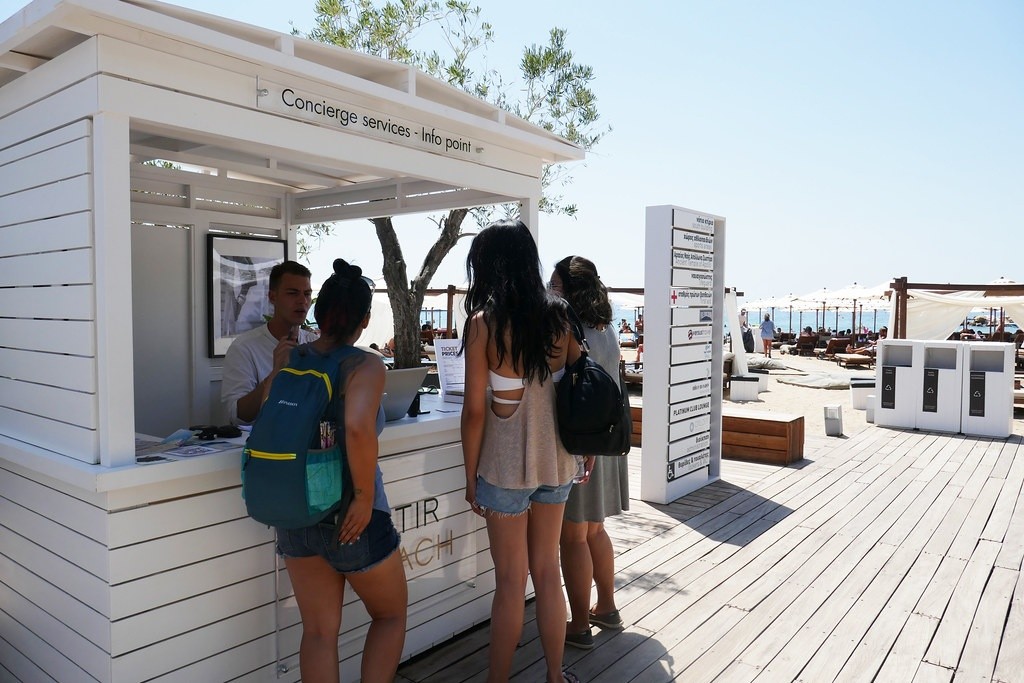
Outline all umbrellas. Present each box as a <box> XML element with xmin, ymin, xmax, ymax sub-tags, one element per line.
<box><xmin>743</xmin><ymin>278</ymin><xmax>919</xmax><ymax>350</ymax></box>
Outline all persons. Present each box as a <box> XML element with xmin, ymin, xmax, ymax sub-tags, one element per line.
<box><xmin>845</xmin><ymin>326</ymin><xmax>888</xmax><ymax>353</ymax></box>
<box><xmin>369</xmin><ymin>343</ymin><xmax>394</xmax><ymax>357</ymax></box>
<box><xmin>960</xmin><ymin>324</ymin><xmax>1001</xmax><ymax>340</ymax></box>
<box><xmin>739</xmin><ymin>308</ymin><xmax>748</xmax><ymax>333</ymax></box>
<box><xmin>459</xmin><ymin>217</ymin><xmax>594</xmax><ymax>683</ymax></box>
<box><xmin>273</xmin><ymin>258</ymin><xmax>409</xmax><ymax>683</ymax></box>
<box><xmin>421</xmin><ymin>325</ymin><xmax>436</xmax><ymax>346</ymax></box>
<box><xmin>777</xmin><ymin>328</ymin><xmax>784</xmax><ymax>337</ymax></box>
<box><xmin>619</xmin><ymin>315</ymin><xmax>642</xmax><ymax>333</ymax></box>
<box><xmin>760</xmin><ymin>313</ymin><xmax>775</xmax><ymax>358</ymax></box>
<box><xmin>219</xmin><ymin>261</ymin><xmax>318</xmax><ymax>428</ymax></box>
<box><xmin>545</xmin><ymin>255</ymin><xmax>630</xmax><ymax>650</ymax></box>
<box><xmin>801</xmin><ymin>326</ymin><xmax>815</xmax><ymax>337</ymax></box>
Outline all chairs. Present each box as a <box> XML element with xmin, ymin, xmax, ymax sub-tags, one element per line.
<box><xmin>772</xmin><ymin>332</ymin><xmax>877</xmax><ymax>368</ymax></box>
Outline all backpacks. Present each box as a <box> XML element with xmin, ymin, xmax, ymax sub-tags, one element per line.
<box><xmin>240</xmin><ymin>345</ymin><xmax>362</xmax><ymax>529</ymax></box>
<box><xmin>555</xmin><ymin>296</ymin><xmax>631</xmax><ymax>456</ymax></box>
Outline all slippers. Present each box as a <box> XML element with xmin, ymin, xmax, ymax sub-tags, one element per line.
<box><xmin>561</xmin><ymin>670</ymin><xmax>580</xmax><ymax>683</ymax></box>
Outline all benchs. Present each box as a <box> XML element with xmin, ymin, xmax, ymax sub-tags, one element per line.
<box><xmin>630</xmin><ymin>401</ymin><xmax>804</xmax><ymax>466</ymax></box>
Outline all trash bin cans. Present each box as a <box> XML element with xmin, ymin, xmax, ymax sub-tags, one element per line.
<box><xmin>874</xmin><ymin>338</ymin><xmax>1016</xmax><ymax>439</ymax></box>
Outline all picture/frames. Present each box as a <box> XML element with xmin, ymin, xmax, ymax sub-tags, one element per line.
<box><xmin>207</xmin><ymin>233</ymin><xmax>288</xmax><ymax>358</ymax></box>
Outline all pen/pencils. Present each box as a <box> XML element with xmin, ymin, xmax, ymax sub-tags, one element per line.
<box><xmin>287</xmin><ymin>325</ymin><xmax>294</xmax><ymax>340</ymax></box>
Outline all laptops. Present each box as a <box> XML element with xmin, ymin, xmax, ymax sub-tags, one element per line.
<box><xmin>381</xmin><ymin>367</ymin><xmax>430</xmax><ymax>422</ymax></box>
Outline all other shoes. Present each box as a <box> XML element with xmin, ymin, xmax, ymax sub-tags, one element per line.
<box><xmin>564</xmin><ymin>619</ymin><xmax>595</xmax><ymax>648</ymax></box>
<box><xmin>586</xmin><ymin>604</ymin><xmax>624</xmax><ymax>628</ymax></box>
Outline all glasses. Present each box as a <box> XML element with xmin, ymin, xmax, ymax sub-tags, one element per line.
<box><xmin>548</xmin><ymin>282</ymin><xmax>563</xmax><ymax>291</ymax></box>
<box><xmin>360</xmin><ymin>276</ymin><xmax>375</xmax><ymax>320</ymax></box>
